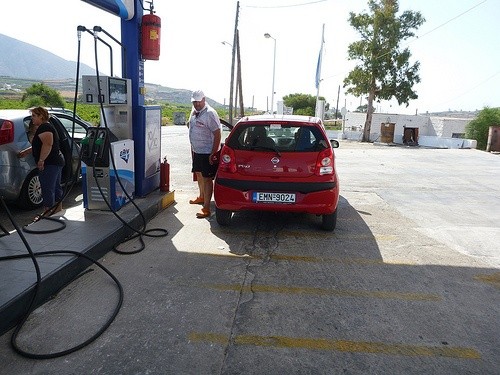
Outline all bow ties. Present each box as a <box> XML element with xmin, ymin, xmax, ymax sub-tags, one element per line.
<box><xmin>192</xmin><ymin>110</ymin><xmax>200</xmax><ymax>117</ymax></box>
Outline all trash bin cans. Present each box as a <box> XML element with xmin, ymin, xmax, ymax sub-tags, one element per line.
<box><xmin>381</xmin><ymin>123</ymin><xmax>396</xmax><ymax>143</ymax></box>
<box><xmin>403</xmin><ymin>124</ymin><xmax>419</xmax><ymax>147</ymax></box>
<box><xmin>174</xmin><ymin>112</ymin><xmax>186</xmax><ymax>125</ymax></box>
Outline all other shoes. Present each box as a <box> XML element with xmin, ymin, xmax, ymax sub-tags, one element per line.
<box><xmin>35</xmin><ymin>207</ymin><xmax>51</xmax><ymax>220</ymax></box>
<box><xmin>54</xmin><ymin>202</ymin><xmax>62</xmax><ymax>212</ymax></box>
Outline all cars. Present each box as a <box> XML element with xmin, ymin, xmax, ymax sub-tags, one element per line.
<box><xmin>192</xmin><ymin>114</ymin><xmax>341</xmax><ymax>231</ymax></box>
<box><xmin>0</xmin><ymin>106</ymin><xmax>93</xmax><ymax>209</ymax></box>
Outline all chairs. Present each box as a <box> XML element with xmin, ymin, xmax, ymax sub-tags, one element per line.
<box><xmin>245</xmin><ymin>128</ymin><xmax>275</xmax><ymax>148</ymax></box>
<box><xmin>289</xmin><ymin>127</ymin><xmax>316</xmax><ymax>148</ymax></box>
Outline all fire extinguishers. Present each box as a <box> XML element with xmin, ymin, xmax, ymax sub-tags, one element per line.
<box><xmin>160</xmin><ymin>155</ymin><xmax>169</xmax><ymax>192</ymax></box>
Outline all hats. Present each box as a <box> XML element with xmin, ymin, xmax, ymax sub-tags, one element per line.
<box><xmin>190</xmin><ymin>90</ymin><xmax>205</xmax><ymax>102</ymax></box>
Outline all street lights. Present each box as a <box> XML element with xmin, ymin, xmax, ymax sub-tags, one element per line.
<box><xmin>222</xmin><ymin>40</ymin><xmax>238</xmax><ymax>117</ymax></box>
<box><xmin>264</xmin><ymin>33</ymin><xmax>277</xmax><ymax>114</ymax></box>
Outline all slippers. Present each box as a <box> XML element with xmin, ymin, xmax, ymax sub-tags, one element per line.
<box><xmin>189</xmin><ymin>197</ymin><xmax>204</xmax><ymax>204</ymax></box>
<box><xmin>196</xmin><ymin>208</ymin><xmax>211</xmax><ymax>217</ymax></box>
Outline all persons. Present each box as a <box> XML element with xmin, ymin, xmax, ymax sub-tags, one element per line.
<box><xmin>26</xmin><ymin>120</ymin><xmax>38</xmax><ymax>143</ymax></box>
<box><xmin>30</xmin><ymin>106</ymin><xmax>65</xmax><ymax>221</ymax></box>
<box><xmin>188</xmin><ymin>89</ymin><xmax>222</xmax><ymax>217</ymax></box>
